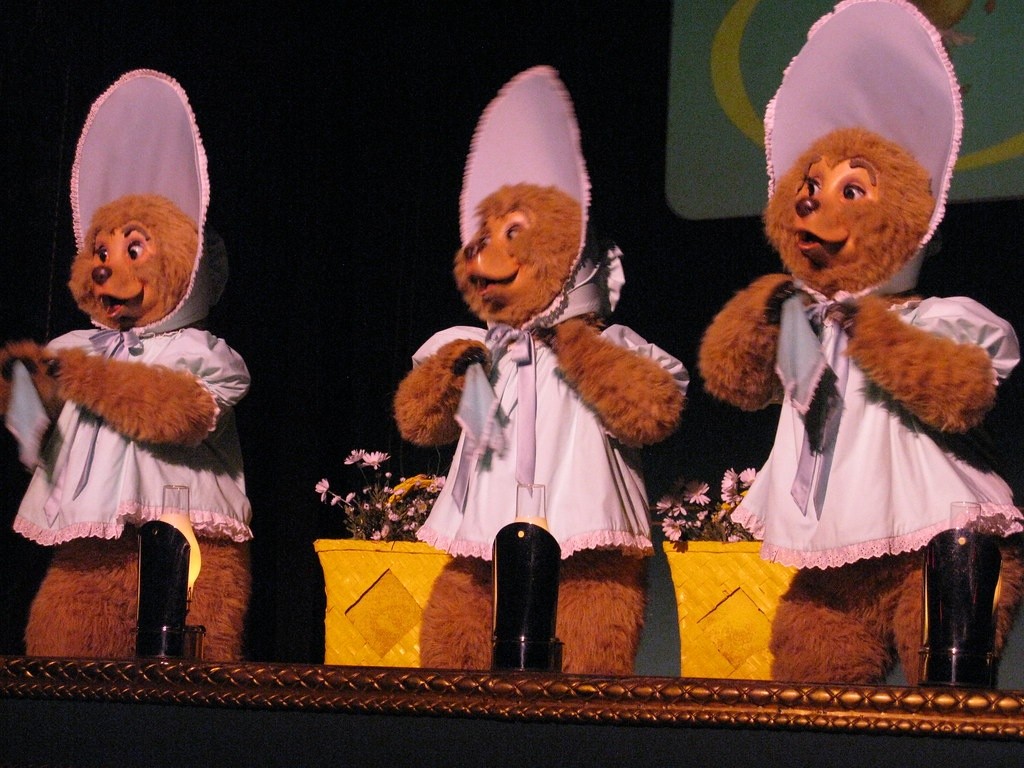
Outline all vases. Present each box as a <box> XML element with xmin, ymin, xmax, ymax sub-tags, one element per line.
<box><xmin>313</xmin><ymin>539</ymin><xmax>491</xmax><ymax>670</ymax></box>
<box><xmin>663</xmin><ymin>540</ymin><xmax>799</xmax><ymax>682</ymax></box>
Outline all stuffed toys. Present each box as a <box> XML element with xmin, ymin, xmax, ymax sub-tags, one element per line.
<box><xmin>0</xmin><ymin>70</ymin><xmax>254</xmax><ymax>671</ymax></box>
<box><xmin>395</xmin><ymin>63</ymin><xmax>690</xmax><ymax>674</ymax></box>
<box><xmin>699</xmin><ymin>1</ymin><xmax>1022</xmax><ymax>688</ymax></box>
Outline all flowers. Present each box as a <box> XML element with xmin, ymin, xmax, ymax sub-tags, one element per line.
<box><xmin>315</xmin><ymin>448</ymin><xmax>446</xmax><ymax>541</ymax></box>
<box><xmin>655</xmin><ymin>461</ymin><xmax>756</xmax><ymax>544</ymax></box>
